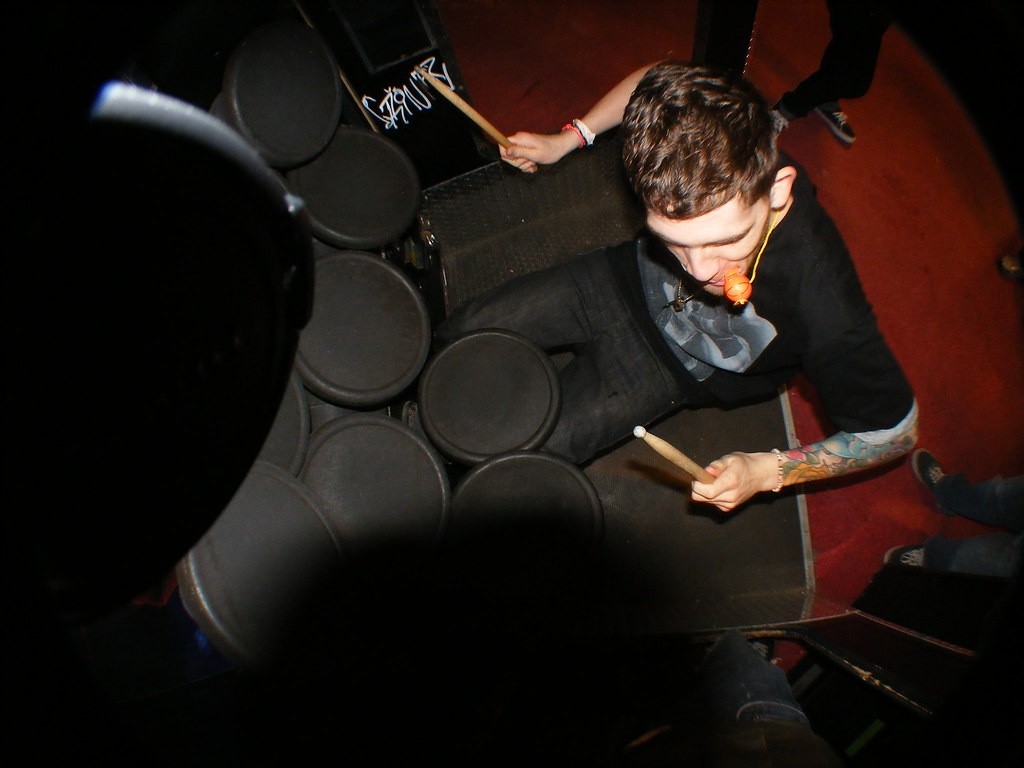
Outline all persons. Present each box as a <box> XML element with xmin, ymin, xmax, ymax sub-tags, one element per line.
<box><xmin>767</xmin><ymin>0</ymin><xmax>889</xmax><ymax>143</ymax></box>
<box><xmin>438</xmin><ymin>60</ymin><xmax>919</xmax><ymax>511</ymax></box>
<box><xmin>884</xmin><ymin>449</ymin><xmax>1024</xmax><ymax>578</ymax></box>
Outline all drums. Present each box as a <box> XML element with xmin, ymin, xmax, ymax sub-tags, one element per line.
<box><xmin>171</xmin><ymin>21</ymin><xmax>608</xmax><ymax>678</ymax></box>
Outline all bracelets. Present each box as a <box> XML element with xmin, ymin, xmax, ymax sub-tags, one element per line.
<box><xmin>563</xmin><ymin>119</ymin><xmax>596</xmax><ymax>148</ymax></box>
<box><xmin>771</xmin><ymin>449</ymin><xmax>783</xmax><ymax>492</ymax></box>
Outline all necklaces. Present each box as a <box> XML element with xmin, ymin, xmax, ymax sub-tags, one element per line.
<box><xmin>675</xmin><ymin>270</ymin><xmax>700</xmax><ymax>308</ymax></box>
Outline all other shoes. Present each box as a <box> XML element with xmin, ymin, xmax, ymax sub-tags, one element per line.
<box><xmin>997</xmin><ymin>252</ymin><xmax>1023</xmax><ymax>278</ymax></box>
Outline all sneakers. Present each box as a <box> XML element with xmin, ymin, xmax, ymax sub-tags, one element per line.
<box><xmin>819</xmin><ymin>103</ymin><xmax>855</xmax><ymax>144</ymax></box>
<box><xmin>770</xmin><ymin>98</ymin><xmax>789</xmax><ymax>140</ymax></box>
<box><xmin>883</xmin><ymin>544</ymin><xmax>923</xmax><ymax>568</ymax></box>
<box><xmin>912</xmin><ymin>448</ymin><xmax>956</xmax><ymax>515</ymax></box>
